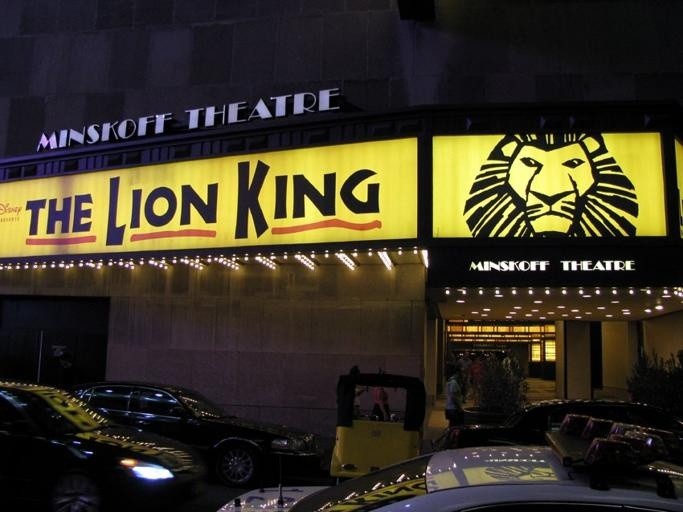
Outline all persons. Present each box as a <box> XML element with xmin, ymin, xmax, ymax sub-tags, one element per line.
<box><xmin>348</xmin><ymin>364</ymin><xmax>367</xmax><ymax>420</ymax></box>
<box><xmin>454</xmin><ymin>351</ymin><xmax>520</xmax><ymax>406</ymax></box>
<box><xmin>443</xmin><ymin>363</ymin><xmax>466</xmax><ymax>430</ymax></box>
<box><xmin>370</xmin><ymin>369</ymin><xmax>393</xmax><ymax>421</ymax></box>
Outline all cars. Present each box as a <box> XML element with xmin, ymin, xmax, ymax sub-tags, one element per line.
<box><xmin>66</xmin><ymin>381</ymin><xmax>325</xmax><ymax>489</ymax></box>
<box><xmin>214</xmin><ymin>412</ymin><xmax>683</xmax><ymax>512</ymax></box>
<box><xmin>429</xmin><ymin>398</ymin><xmax>683</xmax><ymax>468</ymax></box>
<box><xmin>0</xmin><ymin>380</ymin><xmax>208</xmax><ymax>512</ymax></box>
